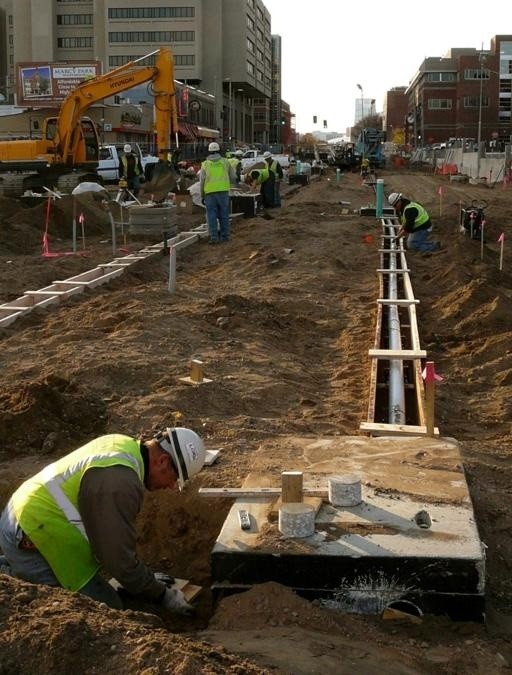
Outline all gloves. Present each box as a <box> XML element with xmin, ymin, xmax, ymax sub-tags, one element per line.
<box><xmin>153</xmin><ymin>572</ymin><xmax>197</xmax><ymax>616</ymax></box>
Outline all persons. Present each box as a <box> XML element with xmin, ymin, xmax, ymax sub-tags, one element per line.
<box><xmin>0</xmin><ymin>425</ymin><xmax>207</xmax><ymax>624</ymax></box>
<box><xmin>118</xmin><ymin>144</ymin><xmax>145</xmax><ymax>201</ymax></box>
<box><xmin>199</xmin><ymin>142</ymin><xmax>238</xmax><ymax>245</ymax></box>
<box><xmin>387</xmin><ymin>192</ymin><xmax>441</xmax><ymax>253</ymax></box>
<box><xmin>219</xmin><ymin>139</ymin><xmax>285</xmax><ymax>210</ymax></box>
<box><xmin>142</xmin><ymin>150</ymin><xmax>203</xmax><ymax>180</ymax></box>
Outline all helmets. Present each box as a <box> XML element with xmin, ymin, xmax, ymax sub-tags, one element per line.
<box><xmin>123</xmin><ymin>144</ymin><xmax>132</xmax><ymax>153</ymax></box>
<box><xmin>235</xmin><ymin>150</ymin><xmax>244</xmax><ymax>157</ymax></box>
<box><xmin>208</xmin><ymin>142</ymin><xmax>220</xmax><ymax>152</ymax></box>
<box><xmin>263</xmin><ymin>151</ymin><xmax>273</xmax><ymax>159</ymax></box>
<box><xmin>164</xmin><ymin>426</ymin><xmax>206</xmax><ymax>494</ymax></box>
<box><xmin>388</xmin><ymin>192</ymin><xmax>403</xmax><ymax>206</ymax></box>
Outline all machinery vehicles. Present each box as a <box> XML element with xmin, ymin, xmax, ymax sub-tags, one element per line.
<box><xmin>0</xmin><ymin>47</ymin><xmax>181</xmax><ymax>195</ymax></box>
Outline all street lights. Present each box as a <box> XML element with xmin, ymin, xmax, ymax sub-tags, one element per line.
<box><xmin>476</xmin><ymin>51</ymin><xmax>489</xmax><ymax>180</ymax></box>
<box><xmin>357</xmin><ymin>85</ymin><xmax>365</xmax><ymax>161</ymax></box>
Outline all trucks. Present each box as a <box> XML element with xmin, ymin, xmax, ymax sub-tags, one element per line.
<box><xmin>96</xmin><ymin>144</ymin><xmax>159</xmax><ymax>183</ymax></box>
<box><xmin>240</xmin><ymin>150</ymin><xmax>290</xmax><ymax>172</ymax></box>
